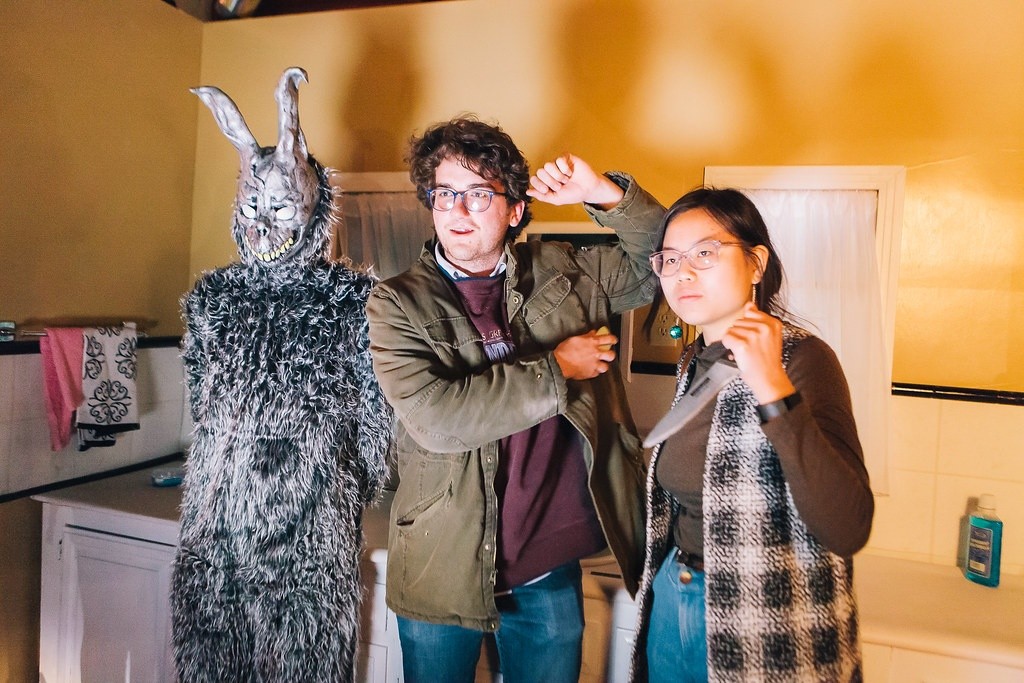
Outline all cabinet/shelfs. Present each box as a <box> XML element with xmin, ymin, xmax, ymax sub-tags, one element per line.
<box><xmin>35</xmin><ymin>503</ymin><xmax>1024</xmax><ymax>683</ymax></box>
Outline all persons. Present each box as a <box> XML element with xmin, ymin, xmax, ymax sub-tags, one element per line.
<box><xmin>366</xmin><ymin>114</ymin><xmax>669</xmax><ymax>683</ymax></box>
<box><xmin>626</xmin><ymin>186</ymin><xmax>875</xmax><ymax>682</ymax></box>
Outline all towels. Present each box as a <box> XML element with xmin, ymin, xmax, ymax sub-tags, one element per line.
<box><xmin>39</xmin><ymin>325</ymin><xmax>142</xmax><ymax>453</ymax></box>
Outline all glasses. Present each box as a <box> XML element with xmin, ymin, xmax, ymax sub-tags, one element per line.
<box><xmin>647</xmin><ymin>240</ymin><xmax>746</xmax><ymax>279</ymax></box>
<box><xmin>426</xmin><ymin>188</ymin><xmax>517</xmax><ymax>213</ymax></box>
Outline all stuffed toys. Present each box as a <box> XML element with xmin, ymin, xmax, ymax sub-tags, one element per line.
<box><xmin>170</xmin><ymin>65</ymin><xmax>398</xmax><ymax>683</ymax></box>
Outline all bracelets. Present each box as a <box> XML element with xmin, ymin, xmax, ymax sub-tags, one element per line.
<box><xmin>752</xmin><ymin>391</ymin><xmax>804</xmax><ymax>425</ymax></box>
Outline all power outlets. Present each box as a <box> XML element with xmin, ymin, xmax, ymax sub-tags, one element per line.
<box><xmin>649</xmin><ymin>303</ymin><xmax>679</xmax><ymax>346</ymax></box>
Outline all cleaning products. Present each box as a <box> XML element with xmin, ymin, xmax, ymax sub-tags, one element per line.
<box><xmin>962</xmin><ymin>491</ymin><xmax>1004</xmax><ymax>588</ymax></box>
